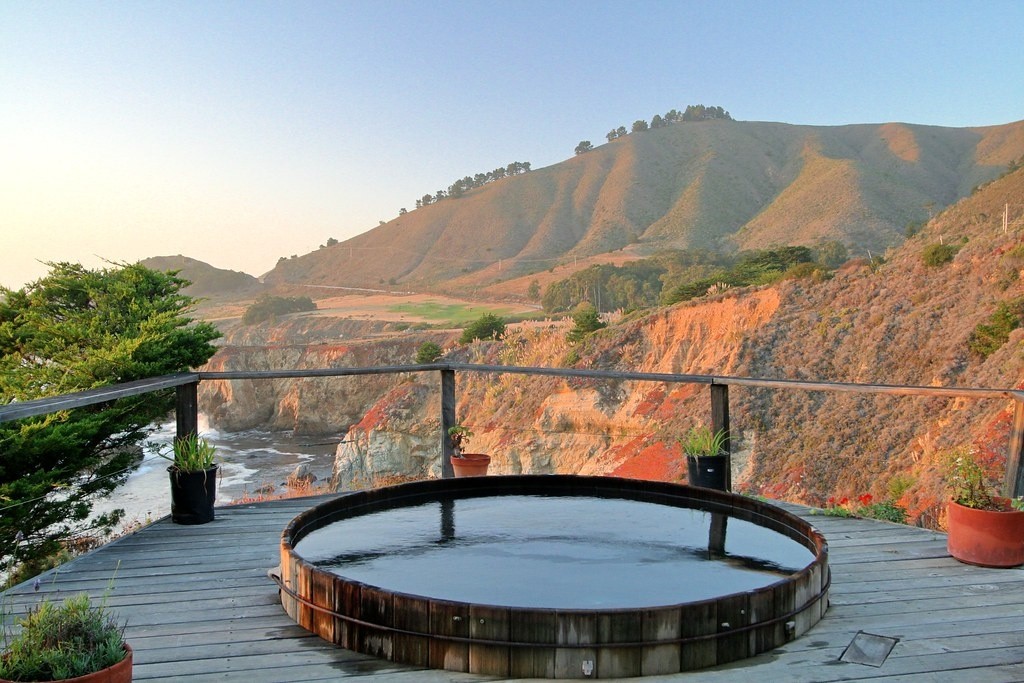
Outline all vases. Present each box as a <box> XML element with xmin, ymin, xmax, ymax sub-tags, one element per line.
<box><xmin>948</xmin><ymin>445</ymin><xmax>1024</xmax><ymax>569</ymax></box>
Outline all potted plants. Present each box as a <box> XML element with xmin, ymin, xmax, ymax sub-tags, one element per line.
<box><xmin>675</xmin><ymin>424</ymin><xmax>743</xmax><ymax>492</ymax></box>
<box><xmin>145</xmin><ymin>428</ymin><xmax>221</xmax><ymax>525</ymax></box>
<box><xmin>0</xmin><ymin>557</ymin><xmax>134</xmax><ymax>683</ymax></box>
<box><xmin>448</xmin><ymin>425</ymin><xmax>491</xmax><ymax>479</ymax></box>
